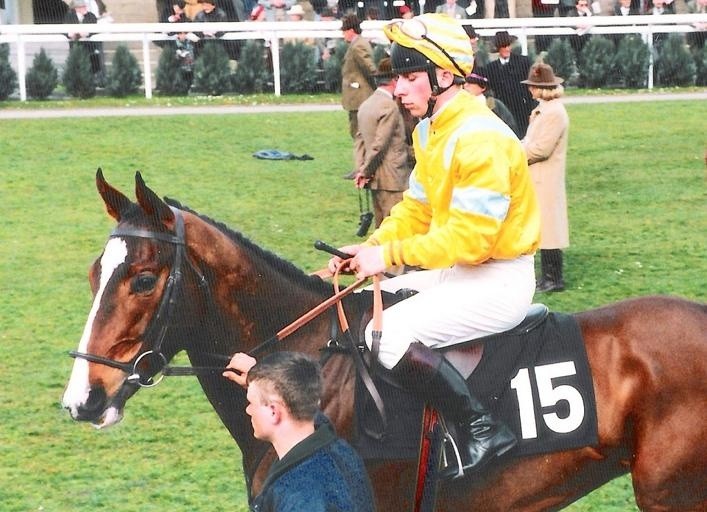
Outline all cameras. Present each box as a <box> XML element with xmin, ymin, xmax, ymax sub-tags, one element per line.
<box><xmin>356</xmin><ymin>213</ymin><xmax>374</xmax><ymax>237</ymax></box>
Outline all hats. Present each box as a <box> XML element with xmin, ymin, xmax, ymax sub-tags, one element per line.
<box><xmin>399</xmin><ymin>5</ymin><xmax>411</xmax><ymax>15</ymax></box>
<box><xmin>489</xmin><ymin>31</ymin><xmax>518</xmax><ymax>53</ymax></box>
<box><xmin>465</xmin><ymin>66</ymin><xmax>492</xmax><ymax>86</ymax></box>
<box><xmin>321</xmin><ymin>5</ymin><xmax>335</xmax><ymax>17</ymax></box>
<box><xmin>460</xmin><ymin>24</ymin><xmax>480</xmax><ymax>40</ymax></box>
<box><xmin>286</xmin><ymin>4</ymin><xmax>306</xmax><ymax>16</ymax></box>
<box><xmin>68</xmin><ymin>0</ymin><xmax>87</xmax><ymax>10</ymax></box>
<box><xmin>340</xmin><ymin>13</ymin><xmax>363</xmax><ymax>30</ymax></box>
<box><xmin>518</xmin><ymin>63</ymin><xmax>565</xmax><ymax>86</ymax></box>
<box><xmin>371</xmin><ymin>57</ymin><xmax>391</xmax><ymax>77</ymax></box>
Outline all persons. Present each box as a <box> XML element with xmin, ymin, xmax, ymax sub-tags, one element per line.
<box><xmin>223</xmin><ymin>353</ymin><xmax>380</xmax><ymax>508</ymax></box>
<box><xmin>155</xmin><ymin>0</ymin><xmax>227</xmax><ymax>71</ymax></box>
<box><xmin>645</xmin><ymin>1</ymin><xmax>707</xmax><ymax>52</ymax></box>
<box><xmin>560</xmin><ymin>0</ymin><xmax>597</xmax><ymax>54</ymax></box>
<box><xmin>438</xmin><ymin>0</ymin><xmax>467</xmax><ymax>19</ymax></box>
<box><xmin>86</xmin><ymin>1</ymin><xmax>108</xmax><ymax>82</ymax></box>
<box><xmin>341</xmin><ymin>14</ymin><xmax>378</xmax><ymax>180</ymax></box>
<box><xmin>351</xmin><ymin>58</ymin><xmax>407</xmax><ymax>229</ymax></box>
<box><xmin>61</xmin><ymin>1</ymin><xmax>100</xmax><ymax>78</ymax></box>
<box><xmin>532</xmin><ymin>0</ymin><xmax>557</xmax><ymax>54</ymax></box>
<box><xmin>399</xmin><ymin>7</ymin><xmax>412</xmax><ymax>19</ymax></box>
<box><xmin>255</xmin><ymin>1</ymin><xmax>341</xmax><ymax>67</ymax></box>
<box><xmin>486</xmin><ymin>30</ymin><xmax>534</xmax><ymax>127</ymax></box>
<box><xmin>461</xmin><ymin>24</ymin><xmax>480</xmax><ymax>88</ymax></box>
<box><xmin>464</xmin><ymin>68</ymin><xmax>516</xmax><ymax>131</ymax></box>
<box><xmin>518</xmin><ymin>63</ymin><xmax>570</xmax><ymax>294</ymax></box>
<box><xmin>613</xmin><ymin>1</ymin><xmax>637</xmax><ymax>41</ymax></box>
<box><xmin>364</xmin><ymin>7</ymin><xmax>379</xmax><ymax>25</ymax></box>
<box><xmin>329</xmin><ymin>11</ymin><xmax>540</xmax><ymax>483</ymax></box>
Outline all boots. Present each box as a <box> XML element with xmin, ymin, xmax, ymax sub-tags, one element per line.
<box><xmin>535</xmin><ymin>249</ymin><xmax>564</xmax><ymax>292</ymax></box>
<box><xmin>391</xmin><ymin>340</ymin><xmax>519</xmax><ymax>482</ymax></box>
<box><xmin>537</xmin><ymin>248</ymin><xmax>546</xmax><ymax>288</ymax></box>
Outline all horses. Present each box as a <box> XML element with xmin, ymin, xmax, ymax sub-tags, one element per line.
<box><xmin>61</xmin><ymin>165</ymin><xmax>706</xmax><ymax>512</ymax></box>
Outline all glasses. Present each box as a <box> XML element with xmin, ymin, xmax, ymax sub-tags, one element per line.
<box><xmin>577</xmin><ymin>3</ymin><xmax>588</xmax><ymax>6</ymax></box>
